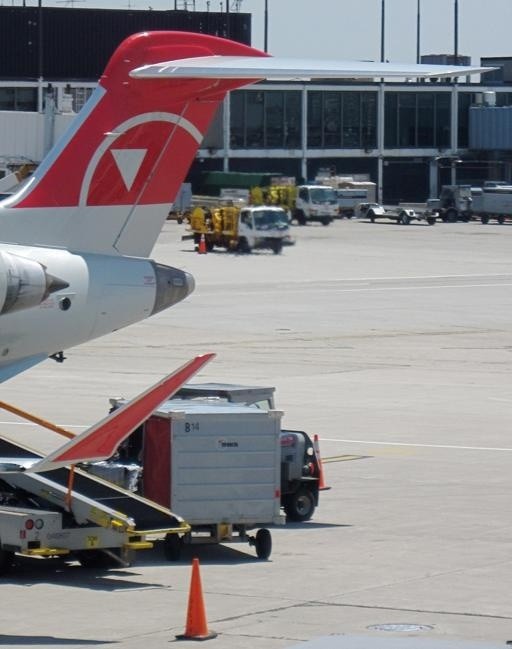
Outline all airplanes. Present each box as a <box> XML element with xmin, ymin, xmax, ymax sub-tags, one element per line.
<box><xmin>0</xmin><ymin>27</ymin><xmax>503</xmax><ymax>478</ymax></box>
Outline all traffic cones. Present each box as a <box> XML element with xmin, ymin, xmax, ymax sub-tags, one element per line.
<box><xmin>175</xmin><ymin>558</ymin><xmax>217</xmax><ymax>641</ymax></box>
<box><xmin>198</xmin><ymin>233</ymin><xmax>207</xmax><ymax>255</ymax></box>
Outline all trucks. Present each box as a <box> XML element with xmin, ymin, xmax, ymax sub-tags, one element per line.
<box><xmin>249</xmin><ymin>183</ymin><xmax>340</xmax><ymax>226</ymax></box>
<box><xmin>181</xmin><ymin>206</ymin><xmax>295</xmax><ymax>255</ymax></box>
